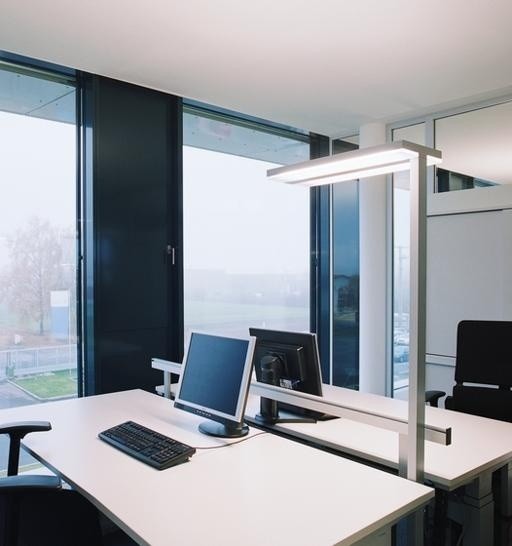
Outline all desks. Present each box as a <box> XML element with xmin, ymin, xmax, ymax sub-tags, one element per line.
<box><xmin>151</xmin><ymin>369</ymin><xmax>511</xmax><ymax>543</ymax></box>
<box><xmin>0</xmin><ymin>384</ymin><xmax>439</xmax><ymax>545</ymax></box>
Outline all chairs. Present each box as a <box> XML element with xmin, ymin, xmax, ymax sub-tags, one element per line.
<box><xmin>419</xmin><ymin>316</ymin><xmax>512</xmax><ymax>421</ymax></box>
<box><xmin>0</xmin><ymin>419</ymin><xmax>107</xmax><ymax>543</ymax></box>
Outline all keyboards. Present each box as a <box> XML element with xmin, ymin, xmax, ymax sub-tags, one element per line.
<box><xmin>98</xmin><ymin>421</ymin><xmax>196</xmax><ymax>470</ymax></box>
<box><xmin>278</xmin><ymin>402</ymin><xmax>340</xmax><ymax>421</ymax></box>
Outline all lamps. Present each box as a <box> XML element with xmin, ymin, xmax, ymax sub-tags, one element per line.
<box><xmin>261</xmin><ymin>138</ymin><xmax>442</xmax><ymax>191</ymax></box>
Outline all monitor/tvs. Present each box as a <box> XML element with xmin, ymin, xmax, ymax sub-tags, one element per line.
<box><xmin>249</xmin><ymin>328</ymin><xmax>323</xmax><ymax>423</ymax></box>
<box><xmin>174</xmin><ymin>327</ymin><xmax>257</xmax><ymax>437</ymax></box>
